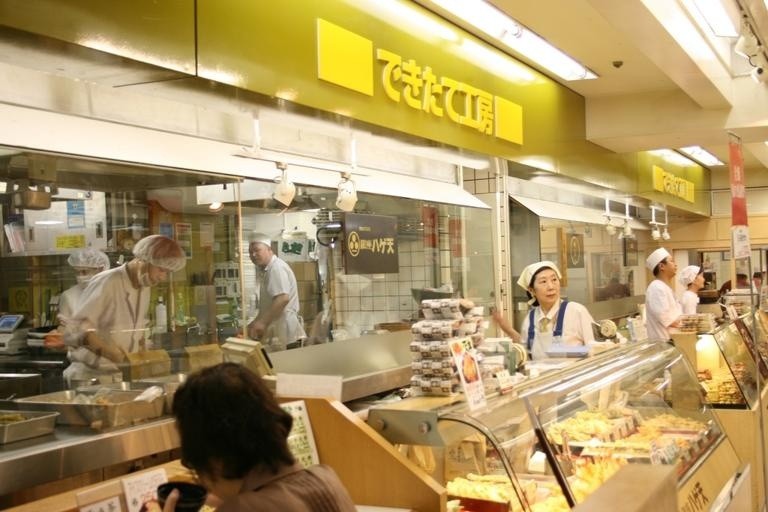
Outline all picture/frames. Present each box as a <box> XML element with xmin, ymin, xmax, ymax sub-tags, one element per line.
<box><xmin>722</xmin><ymin>251</ymin><xmax>731</xmax><ymax>261</ymax></box>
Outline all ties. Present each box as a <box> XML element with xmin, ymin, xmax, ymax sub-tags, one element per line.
<box><xmin>539</xmin><ymin>318</ymin><xmax>552</xmax><ymax>335</ymax></box>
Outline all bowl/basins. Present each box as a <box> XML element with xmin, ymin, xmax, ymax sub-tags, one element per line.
<box><xmin>155</xmin><ymin>480</ymin><xmax>204</xmax><ymax>510</ymax></box>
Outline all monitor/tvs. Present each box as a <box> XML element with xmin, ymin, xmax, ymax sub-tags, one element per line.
<box><xmin>0</xmin><ymin>314</ymin><xmax>23</xmax><ymax>331</ymax></box>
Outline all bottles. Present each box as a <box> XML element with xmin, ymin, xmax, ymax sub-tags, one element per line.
<box><xmin>155</xmin><ymin>296</ymin><xmax>169</xmax><ymax>333</ymax></box>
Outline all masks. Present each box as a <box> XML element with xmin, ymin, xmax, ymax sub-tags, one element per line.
<box><xmin>137</xmin><ymin>260</ymin><xmax>160</xmax><ymax>289</ymax></box>
<box><xmin>72</xmin><ymin>272</ymin><xmax>96</xmax><ymax>288</ymax></box>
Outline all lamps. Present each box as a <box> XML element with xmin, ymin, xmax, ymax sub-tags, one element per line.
<box><xmin>600</xmin><ymin>185</ymin><xmax>636</xmax><ymax>239</ymax></box>
<box><xmin>233</xmin><ymin>153</ymin><xmax>371</xmax><ymax>213</ymax></box>
<box><xmin>647</xmin><ymin>205</ymin><xmax>674</xmax><ymax>242</ymax></box>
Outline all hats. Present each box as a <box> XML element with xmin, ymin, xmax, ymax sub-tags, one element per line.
<box><xmin>643</xmin><ymin>244</ymin><xmax>671</xmax><ymax>274</ymax></box>
<box><xmin>677</xmin><ymin>263</ymin><xmax>703</xmax><ymax>289</ymax></box>
<box><xmin>515</xmin><ymin>259</ymin><xmax>562</xmax><ymax>291</ymax></box>
<box><xmin>247</xmin><ymin>231</ymin><xmax>273</xmax><ymax>248</ymax></box>
<box><xmin>753</xmin><ymin>264</ymin><xmax>766</xmax><ymax>273</ymax></box>
<box><xmin>734</xmin><ymin>266</ymin><xmax>748</xmax><ymax>282</ymax></box>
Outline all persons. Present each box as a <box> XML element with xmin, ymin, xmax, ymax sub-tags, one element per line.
<box><xmin>489</xmin><ymin>261</ymin><xmax>597</xmax><ymax>361</ymax></box>
<box><xmin>48</xmin><ymin>234</ymin><xmax>186</xmax><ymax>387</ymax></box>
<box><xmin>233</xmin><ymin>233</ymin><xmax>306</xmax><ymax>349</ymax></box>
<box><xmin>143</xmin><ymin>362</ymin><xmax>357</xmax><ymax>512</ymax></box>
<box><xmin>644</xmin><ymin>246</ymin><xmax>767</xmax><ymax>345</ymax></box>
